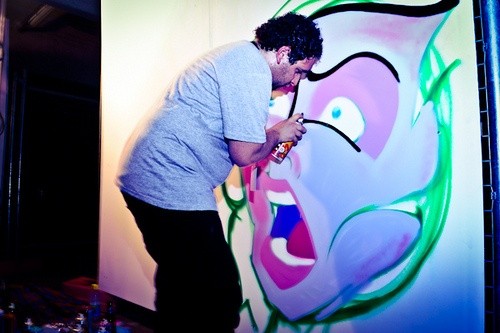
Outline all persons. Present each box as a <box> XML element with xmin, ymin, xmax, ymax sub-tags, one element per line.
<box><xmin>116</xmin><ymin>12</ymin><xmax>325</xmax><ymax>333</ymax></box>
